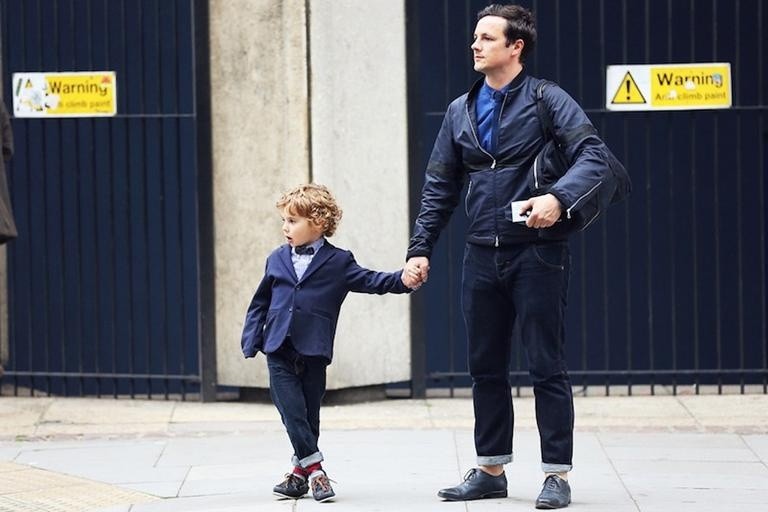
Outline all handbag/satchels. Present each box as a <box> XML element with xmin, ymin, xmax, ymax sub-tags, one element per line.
<box><xmin>528</xmin><ymin>138</ymin><xmax>632</xmax><ymax>240</ymax></box>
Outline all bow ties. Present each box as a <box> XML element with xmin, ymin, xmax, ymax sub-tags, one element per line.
<box><xmin>295</xmin><ymin>246</ymin><xmax>314</xmax><ymax>255</ymax></box>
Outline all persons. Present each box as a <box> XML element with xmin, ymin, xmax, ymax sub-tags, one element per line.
<box><xmin>403</xmin><ymin>4</ymin><xmax>610</xmax><ymax>508</ymax></box>
<box><xmin>239</xmin><ymin>181</ymin><xmax>431</xmax><ymax>503</ymax></box>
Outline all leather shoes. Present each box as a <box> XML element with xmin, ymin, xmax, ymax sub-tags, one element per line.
<box><xmin>535</xmin><ymin>474</ymin><xmax>571</xmax><ymax>509</ymax></box>
<box><xmin>312</xmin><ymin>474</ymin><xmax>336</xmax><ymax>503</ymax></box>
<box><xmin>273</xmin><ymin>472</ymin><xmax>309</xmax><ymax>498</ymax></box>
<box><xmin>438</xmin><ymin>467</ymin><xmax>508</xmax><ymax>501</ymax></box>
